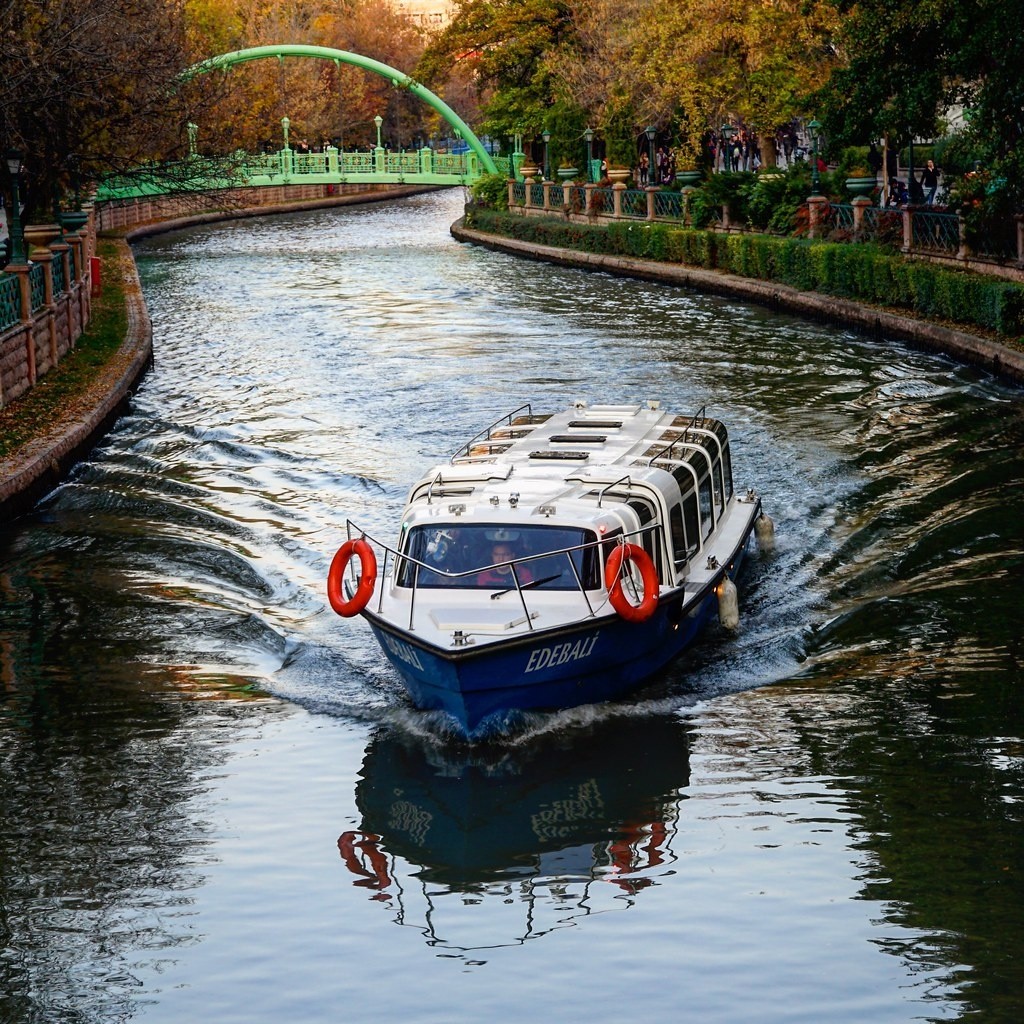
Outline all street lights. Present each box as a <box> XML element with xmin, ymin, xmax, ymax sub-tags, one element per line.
<box><xmin>280</xmin><ymin>117</ymin><xmax>291</xmax><ymax>173</ymax></box>
<box><xmin>806</xmin><ymin>121</ymin><xmax>824</xmax><ymax>197</ymax></box>
<box><xmin>187</xmin><ymin>123</ymin><xmax>198</xmax><ymax>155</ymax></box>
<box><xmin>644</xmin><ymin>126</ymin><xmax>658</xmax><ymax>186</ymax></box>
<box><xmin>541</xmin><ymin>130</ymin><xmax>551</xmax><ymax>181</ymax></box>
<box><xmin>583</xmin><ymin>129</ymin><xmax>595</xmax><ymax>184</ymax></box>
<box><xmin>374</xmin><ymin>115</ymin><xmax>383</xmax><ymax>147</ymax></box>
<box><xmin>722</xmin><ymin>124</ymin><xmax>734</xmax><ymax>173</ymax></box>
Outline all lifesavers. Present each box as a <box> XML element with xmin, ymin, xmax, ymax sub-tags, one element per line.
<box><xmin>604</xmin><ymin>542</ymin><xmax>661</xmax><ymax>624</ymax></box>
<box><xmin>326</xmin><ymin>537</ymin><xmax>378</xmax><ymax>618</ymax></box>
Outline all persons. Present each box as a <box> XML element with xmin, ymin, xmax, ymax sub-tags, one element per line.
<box><xmin>706</xmin><ymin>128</ymin><xmax>801</xmax><ymax>172</ymax></box>
<box><xmin>639</xmin><ymin>148</ymin><xmax>674</xmax><ymax>187</ymax></box>
<box><xmin>884</xmin><ymin>179</ymin><xmax>909</xmax><ymax>209</ymax></box>
<box><xmin>477</xmin><ymin>543</ymin><xmax>534</xmax><ymax>589</ymax></box>
<box><xmin>919</xmin><ymin>158</ymin><xmax>940</xmax><ymax>206</ymax></box>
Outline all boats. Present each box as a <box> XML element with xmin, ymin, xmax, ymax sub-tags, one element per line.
<box><xmin>334</xmin><ymin>716</ymin><xmax>690</xmax><ymax>962</ymax></box>
<box><xmin>328</xmin><ymin>403</ymin><xmax>775</xmax><ymax>728</ymax></box>
